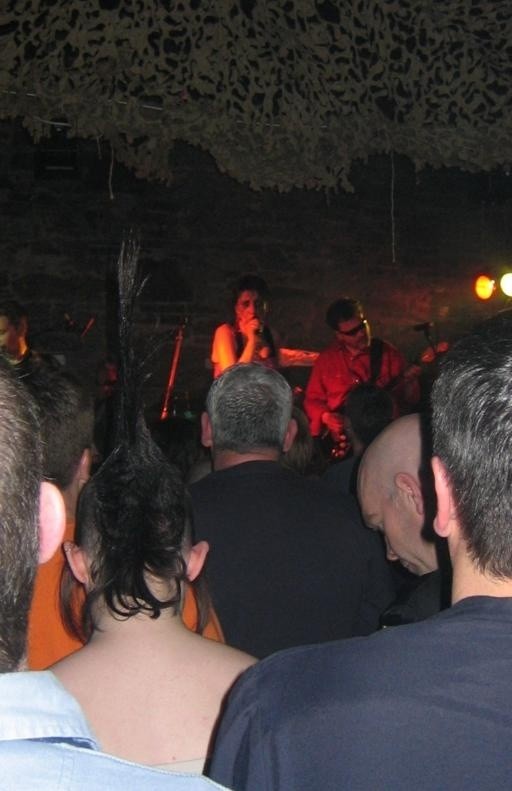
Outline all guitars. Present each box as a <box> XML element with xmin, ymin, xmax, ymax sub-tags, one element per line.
<box><xmin>318</xmin><ymin>341</ymin><xmax>448</xmax><ymax>464</ymax></box>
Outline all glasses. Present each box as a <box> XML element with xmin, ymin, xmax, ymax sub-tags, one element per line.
<box><xmin>340</xmin><ymin>318</ymin><xmax>367</xmax><ymax>336</ymax></box>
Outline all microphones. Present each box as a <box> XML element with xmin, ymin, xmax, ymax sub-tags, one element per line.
<box><xmin>412</xmin><ymin>321</ymin><xmax>435</xmax><ymax>331</ymax></box>
